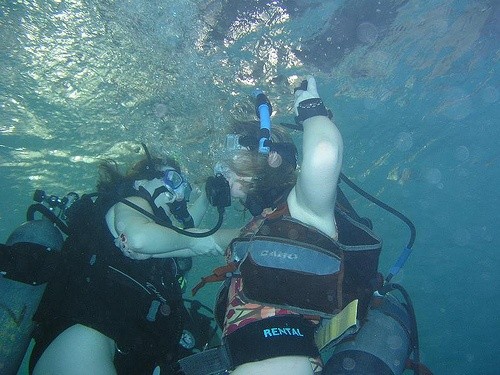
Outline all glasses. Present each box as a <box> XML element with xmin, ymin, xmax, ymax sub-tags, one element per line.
<box><xmin>157</xmin><ymin>169</ymin><xmax>193</xmax><ymax>201</ymax></box>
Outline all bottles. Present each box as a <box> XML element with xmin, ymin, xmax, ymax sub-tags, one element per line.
<box><xmin>0</xmin><ymin>191</ymin><xmax>72</xmax><ymax>375</ymax></box>
<box><xmin>333</xmin><ymin>297</ymin><xmax>414</xmax><ymax>373</ymax></box>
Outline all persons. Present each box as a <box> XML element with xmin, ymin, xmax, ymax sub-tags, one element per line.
<box><xmin>116</xmin><ymin>73</ymin><xmax>383</xmax><ymax>375</ymax></box>
<box><xmin>25</xmin><ymin>143</ymin><xmax>230</xmax><ymax>375</ymax></box>
